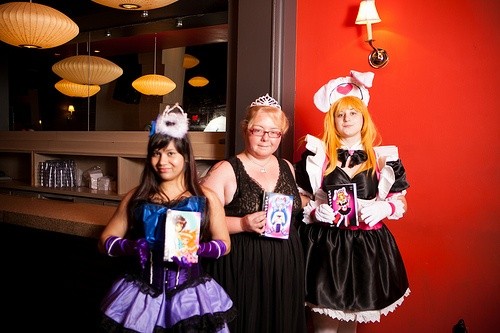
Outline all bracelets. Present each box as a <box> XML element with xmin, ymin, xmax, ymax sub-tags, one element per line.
<box><xmin>108</xmin><ymin>237</ymin><xmax>123</xmax><ymax>257</ymax></box>
<box><xmin>211</xmin><ymin>241</ymin><xmax>221</xmax><ymax>260</ymax></box>
<box><xmin>311</xmin><ymin>208</ymin><xmax>318</xmax><ymax>224</ymax></box>
<box><xmin>389</xmin><ymin>202</ymin><xmax>396</xmax><ymax>217</ymax></box>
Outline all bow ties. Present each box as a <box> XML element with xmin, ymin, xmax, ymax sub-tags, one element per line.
<box><xmin>337</xmin><ymin>149</ymin><xmax>368</xmax><ymax>168</ymax></box>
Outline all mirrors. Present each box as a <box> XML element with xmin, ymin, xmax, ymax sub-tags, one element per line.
<box><xmin>0</xmin><ymin>0</ymin><xmax>230</xmax><ymax>133</ymax></box>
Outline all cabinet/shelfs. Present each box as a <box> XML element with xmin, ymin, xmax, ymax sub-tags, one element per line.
<box><xmin>0</xmin><ymin>146</ymin><xmax>227</xmax><ymax>204</ymax></box>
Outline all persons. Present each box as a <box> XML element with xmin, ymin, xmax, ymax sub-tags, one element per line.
<box><xmin>98</xmin><ymin>102</ymin><xmax>239</xmax><ymax>333</ymax></box>
<box><xmin>199</xmin><ymin>93</ymin><xmax>307</xmax><ymax>333</ymax></box>
<box><xmin>292</xmin><ymin>70</ymin><xmax>410</xmax><ymax>333</ymax></box>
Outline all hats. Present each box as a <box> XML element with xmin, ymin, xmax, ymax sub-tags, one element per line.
<box><xmin>313</xmin><ymin>69</ymin><xmax>374</xmax><ymax>113</ymax></box>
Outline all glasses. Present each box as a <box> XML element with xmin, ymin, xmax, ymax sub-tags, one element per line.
<box><xmin>249</xmin><ymin>129</ymin><xmax>283</xmax><ymax>138</ymax></box>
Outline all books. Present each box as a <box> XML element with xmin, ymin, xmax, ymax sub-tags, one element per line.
<box><xmin>38</xmin><ymin>160</ymin><xmax>77</xmax><ymax>189</ymax></box>
<box><xmin>325</xmin><ymin>183</ymin><xmax>360</xmax><ymax>228</ymax></box>
<box><xmin>260</xmin><ymin>191</ymin><xmax>293</xmax><ymax>240</ymax></box>
<box><xmin>164</xmin><ymin>209</ymin><xmax>201</xmax><ymax>263</ymax></box>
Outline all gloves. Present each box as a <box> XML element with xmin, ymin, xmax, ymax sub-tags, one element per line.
<box><xmin>171</xmin><ymin>240</ymin><xmax>226</xmax><ymax>269</ymax></box>
<box><xmin>301</xmin><ymin>200</ymin><xmax>335</xmax><ymax>224</ymax></box>
<box><xmin>104</xmin><ymin>236</ymin><xmax>148</xmax><ymax>269</ymax></box>
<box><xmin>360</xmin><ymin>200</ymin><xmax>405</xmax><ymax>227</ymax></box>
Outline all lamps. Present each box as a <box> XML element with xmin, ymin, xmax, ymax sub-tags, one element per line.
<box><xmin>354</xmin><ymin>0</ymin><xmax>391</xmax><ymax>69</ymax></box>
<box><xmin>51</xmin><ymin>27</ymin><xmax>123</xmax><ymax>99</ymax></box>
<box><xmin>182</xmin><ymin>54</ymin><xmax>211</xmax><ymax>87</ymax></box>
<box><xmin>0</xmin><ymin>0</ymin><xmax>80</xmax><ymax>50</ymax></box>
<box><xmin>66</xmin><ymin>105</ymin><xmax>75</xmax><ymax>119</ymax></box>
<box><xmin>130</xmin><ymin>33</ymin><xmax>178</xmax><ymax>97</ymax></box>
<box><xmin>91</xmin><ymin>0</ymin><xmax>179</xmax><ymax>11</ymax></box>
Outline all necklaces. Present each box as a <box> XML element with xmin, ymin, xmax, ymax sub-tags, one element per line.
<box><xmin>256</xmin><ymin>161</ymin><xmax>269</xmax><ymax>174</ymax></box>
<box><xmin>341</xmin><ymin>141</ymin><xmax>360</xmax><ymax>155</ymax></box>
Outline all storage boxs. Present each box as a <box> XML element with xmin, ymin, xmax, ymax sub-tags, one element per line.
<box><xmin>87</xmin><ymin>170</ymin><xmax>117</xmax><ymax>191</ymax></box>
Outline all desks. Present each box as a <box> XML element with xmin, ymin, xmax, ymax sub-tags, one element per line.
<box><xmin>0</xmin><ymin>192</ymin><xmax>120</xmax><ymax>239</ymax></box>
